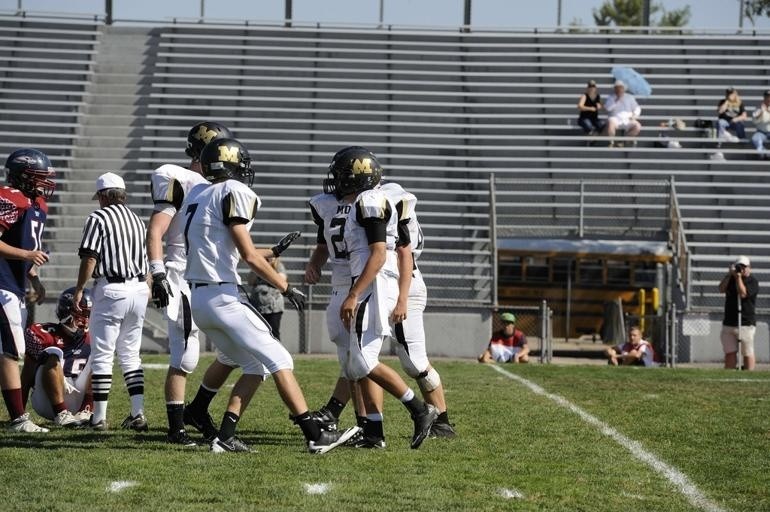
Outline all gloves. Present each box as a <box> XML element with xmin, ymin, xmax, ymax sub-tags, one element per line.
<box><xmin>150</xmin><ymin>273</ymin><xmax>174</xmax><ymax>309</ymax></box>
<box><xmin>272</xmin><ymin>230</ymin><xmax>302</xmax><ymax>258</ymax></box>
<box><xmin>281</xmin><ymin>282</ymin><xmax>307</xmax><ymax>314</ymax></box>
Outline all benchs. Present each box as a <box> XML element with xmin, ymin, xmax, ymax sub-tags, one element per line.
<box><xmin>0</xmin><ymin>13</ymin><xmax>107</xmax><ymax>270</ymax></box>
<box><xmin>529</xmin><ymin>338</ymin><xmax>615</xmax><ymax>357</ymax></box>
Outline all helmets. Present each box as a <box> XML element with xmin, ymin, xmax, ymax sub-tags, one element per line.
<box><xmin>322</xmin><ymin>146</ymin><xmax>383</xmax><ymax>202</ymax></box>
<box><xmin>4</xmin><ymin>149</ymin><xmax>56</xmax><ymax>199</ymax></box>
<box><xmin>201</xmin><ymin>139</ymin><xmax>255</xmax><ymax>187</ymax></box>
<box><xmin>56</xmin><ymin>286</ymin><xmax>94</xmax><ymax>334</ymax></box>
<box><xmin>185</xmin><ymin>120</ymin><xmax>233</xmax><ymax>158</ymax></box>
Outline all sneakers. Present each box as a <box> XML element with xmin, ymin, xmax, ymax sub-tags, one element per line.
<box><xmin>430</xmin><ymin>421</ymin><xmax>458</xmax><ymax>439</ymax></box>
<box><xmin>75</xmin><ymin>409</ymin><xmax>94</xmax><ymax>425</ymax></box>
<box><xmin>183</xmin><ymin>406</ymin><xmax>219</xmax><ymax>438</ymax></box>
<box><xmin>289</xmin><ymin>408</ymin><xmax>340</xmax><ymax>431</ymax></box>
<box><xmin>410</xmin><ymin>402</ymin><xmax>440</xmax><ymax>450</ymax></box>
<box><xmin>304</xmin><ymin>424</ymin><xmax>360</xmax><ymax>454</ymax></box>
<box><xmin>9</xmin><ymin>414</ymin><xmax>51</xmax><ymax>433</ymax></box>
<box><xmin>55</xmin><ymin>409</ymin><xmax>76</xmax><ymax>426</ymax></box>
<box><xmin>90</xmin><ymin>415</ymin><xmax>107</xmax><ymax>431</ymax></box>
<box><xmin>166</xmin><ymin>427</ymin><xmax>197</xmax><ymax>447</ymax></box>
<box><xmin>121</xmin><ymin>414</ymin><xmax>149</xmax><ymax>431</ymax></box>
<box><xmin>343</xmin><ymin>432</ymin><xmax>387</xmax><ymax>449</ymax></box>
<box><xmin>209</xmin><ymin>436</ymin><xmax>258</xmax><ymax>454</ymax></box>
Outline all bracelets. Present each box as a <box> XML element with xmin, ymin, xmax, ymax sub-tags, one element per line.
<box><xmin>725</xmin><ymin>274</ymin><xmax>730</xmax><ymax>279</ymax></box>
<box><xmin>150</xmin><ymin>260</ymin><xmax>166</xmax><ymax>278</ymax></box>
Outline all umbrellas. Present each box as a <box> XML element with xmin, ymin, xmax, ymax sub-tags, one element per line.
<box><xmin>612</xmin><ymin>66</ymin><xmax>652</xmax><ymax>98</ymax></box>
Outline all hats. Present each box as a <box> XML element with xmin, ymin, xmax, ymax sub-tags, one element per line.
<box><xmin>734</xmin><ymin>255</ymin><xmax>750</xmax><ymax>267</ymax></box>
<box><xmin>91</xmin><ymin>171</ymin><xmax>127</xmax><ymax>200</ymax></box>
<box><xmin>499</xmin><ymin>312</ymin><xmax>516</xmax><ymax>323</ymax></box>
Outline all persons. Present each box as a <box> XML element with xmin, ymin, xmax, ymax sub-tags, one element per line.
<box><xmin>306</xmin><ymin>144</ymin><xmax>457</xmax><ymax>449</ymax></box>
<box><xmin>751</xmin><ymin>90</ymin><xmax>770</xmax><ymax>149</ymax></box>
<box><xmin>246</xmin><ymin>255</ymin><xmax>288</xmax><ymax>340</ymax></box>
<box><xmin>578</xmin><ymin>80</ymin><xmax>606</xmax><ymax>144</ymax></box>
<box><xmin>606</xmin><ymin>326</ymin><xmax>654</xmax><ymax>367</ymax></box>
<box><xmin>603</xmin><ymin>81</ymin><xmax>641</xmax><ymax>147</ymax></box>
<box><xmin>73</xmin><ymin>171</ymin><xmax>149</xmax><ymax>432</ymax></box>
<box><xmin>0</xmin><ymin>148</ymin><xmax>55</xmax><ymax>435</ymax></box>
<box><xmin>182</xmin><ymin>137</ymin><xmax>359</xmax><ymax>455</ymax></box>
<box><xmin>478</xmin><ymin>312</ymin><xmax>530</xmax><ymax>364</ymax></box>
<box><xmin>144</xmin><ymin>120</ymin><xmax>238</xmax><ymax>448</ymax></box>
<box><xmin>24</xmin><ymin>286</ymin><xmax>94</xmax><ymax>428</ymax></box>
<box><xmin>719</xmin><ymin>256</ymin><xmax>759</xmax><ymax>370</ymax></box>
<box><xmin>717</xmin><ymin>88</ymin><xmax>748</xmax><ymax>140</ymax></box>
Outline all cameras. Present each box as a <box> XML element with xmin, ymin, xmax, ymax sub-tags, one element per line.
<box><xmin>735</xmin><ymin>264</ymin><xmax>741</xmax><ymax>272</ymax></box>
